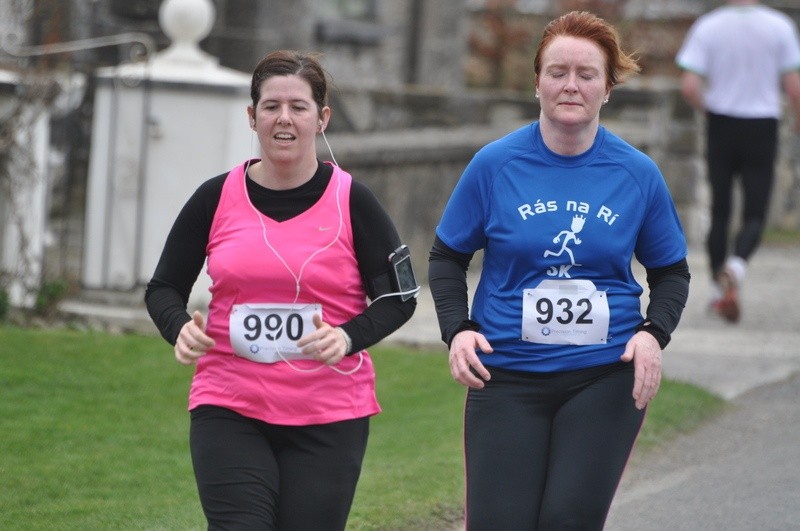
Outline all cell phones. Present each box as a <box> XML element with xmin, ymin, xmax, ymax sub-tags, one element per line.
<box><xmin>387</xmin><ymin>244</ymin><xmax>420</xmax><ymax>304</ymax></box>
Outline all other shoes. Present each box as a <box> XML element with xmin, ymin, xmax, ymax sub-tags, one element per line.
<box><xmin>720</xmin><ymin>267</ymin><xmax>739</xmax><ymax>323</ymax></box>
<box><xmin>706</xmin><ymin>299</ymin><xmax>721</xmax><ymax>313</ymax></box>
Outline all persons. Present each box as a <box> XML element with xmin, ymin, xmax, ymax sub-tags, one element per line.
<box><xmin>427</xmin><ymin>11</ymin><xmax>692</xmax><ymax>531</ymax></box>
<box><xmin>145</xmin><ymin>50</ymin><xmax>417</xmax><ymax>531</ymax></box>
<box><xmin>675</xmin><ymin>0</ymin><xmax>800</xmax><ymax>325</ymax></box>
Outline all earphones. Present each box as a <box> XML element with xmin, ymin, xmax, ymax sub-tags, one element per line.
<box><xmin>251</xmin><ymin>118</ymin><xmax>256</xmax><ymax>129</ymax></box>
<box><xmin>319</xmin><ymin>119</ymin><xmax>324</xmax><ymax>132</ymax></box>
